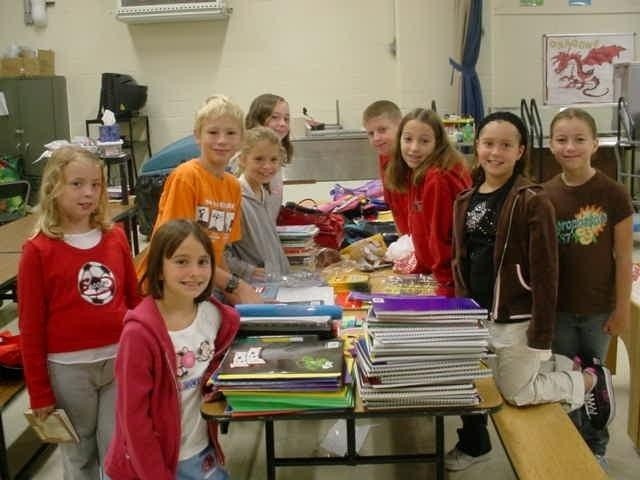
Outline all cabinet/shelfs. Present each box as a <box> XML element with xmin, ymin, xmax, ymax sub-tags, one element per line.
<box><xmin>1</xmin><ymin>76</ymin><xmax>70</xmax><ymax>207</ymax></box>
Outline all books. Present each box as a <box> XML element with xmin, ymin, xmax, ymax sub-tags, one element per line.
<box><xmin>24</xmin><ymin>408</ymin><xmax>80</xmax><ymax>444</ymax></box>
<box><xmin>276</xmin><ymin>224</ymin><xmax>327</xmax><ymax>265</ymax></box>
<box><xmin>350</xmin><ymin>295</ymin><xmax>491</xmax><ymax>410</ymax></box>
<box><xmin>208</xmin><ymin>304</ymin><xmax>357</xmax><ymax>417</ymax></box>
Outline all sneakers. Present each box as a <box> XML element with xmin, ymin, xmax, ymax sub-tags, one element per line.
<box><xmin>445</xmin><ymin>447</ymin><xmax>493</xmax><ymax>471</ymax></box>
<box><xmin>583</xmin><ymin>366</ymin><xmax>615</xmax><ymax>427</ymax></box>
<box><xmin>574</xmin><ymin>354</ymin><xmax>601</xmax><ymax>370</ymax></box>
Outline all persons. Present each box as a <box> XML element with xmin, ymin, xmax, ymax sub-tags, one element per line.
<box><xmin>363</xmin><ymin>101</ymin><xmax>410</xmax><ymax>235</ymax></box>
<box><xmin>384</xmin><ymin>107</ymin><xmax>492</xmax><ymax>472</ymax></box>
<box><xmin>132</xmin><ymin>94</ymin><xmax>263</xmax><ymax>308</ymax></box>
<box><xmin>16</xmin><ymin>146</ymin><xmax>147</xmax><ymax>480</ymax></box>
<box><xmin>103</xmin><ymin>220</ymin><xmax>240</xmax><ymax>480</ymax></box>
<box><xmin>223</xmin><ymin>126</ymin><xmax>290</xmax><ymax>284</ymax></box>
<box><xmin>225</xmin><ymin>93</ymin><xmax>294</xmax><ymax>178</ymax></box>
<box><xmin>451</xmin><ymin>112</ymin><xmax>615</xmax><ymax>429</ymax></box>
<box><xmin>538</xmin><ymin>107</ymin><xmax>634</xmax><ymax>473</ymax></box>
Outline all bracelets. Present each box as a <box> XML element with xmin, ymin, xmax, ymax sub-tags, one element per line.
<box><xmin>225</xmin><ymin>274</ymin><xmax>240</xmax><ymax>293</ymax></box>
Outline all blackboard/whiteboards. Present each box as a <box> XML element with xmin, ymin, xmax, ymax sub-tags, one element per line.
<box><xmin>543</xmin><ymin>32</ymin><xmax>638</xmax><ymax>108</ymax></box>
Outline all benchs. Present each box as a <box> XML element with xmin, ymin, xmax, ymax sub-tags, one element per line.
<box><xmin>486</xmin><ymin>363</ymin><xmax>613</xmax><ymax>479</ymax></box>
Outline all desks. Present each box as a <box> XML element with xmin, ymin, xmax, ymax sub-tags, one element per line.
<box><xmin>0</xmin><ymin>202</ymin><xmax>139</xmax><ymax>480</ymax></box>
<box><xmin>202</xmin><ymin>199</ymin><xmax>504</xmax><ymax>478</ymax></box>
